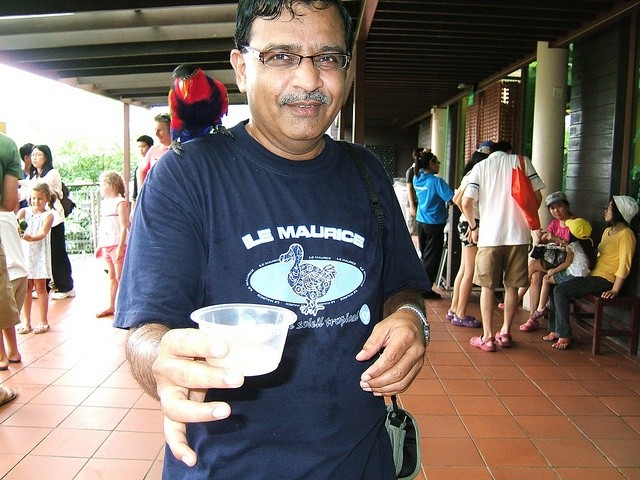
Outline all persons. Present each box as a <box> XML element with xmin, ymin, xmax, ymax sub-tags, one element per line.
<box><xmin>95</xmin><ymin>170</ymin><xmax>128</xmax><ymax>319</ymax></box>
<box><xmin>130</xmin><ymin>134</ymin><xmax>154</xmax><ymax>198</ymax></box>
<box><xmin>446</xmin><ymin>141</ymin><xmax>492</xmax><ymax>328</ymax></box>
<box><xmin>405</xmin><ymin>148</ymin><xmax>431</xmax><ymax>259</ymax></box>
<box><xmin>412</xmin><ymin>148</ymin><xmax>455</xmax><ymax>299</ymax></box>
<box><xmin>1</xmin><ymin>114</ymin><xmax>75</xmax><ymax>403</ymax></box>
<box><xmin>529</xmin><ymin>217</ymin><xmax>597</xmax><ymax>320</ymax></box>
<box><xmin>498</xmin><ymin>191</ymin><xmax>575</xmax><ymax>333</ymax></box>
<box><xmin>542</xmin><ymin>195</ymin><xmax>639</xmax><ymax>349</ymax></box>
<box><xmin>110</xmin><ymin>0</ymin><xmax>432</xmax><ymax>480</ymax></box>
<box><xmin>136</xmin><ymin>113</ymin><xmax>170</xmax><ymax>186</ymax></box>
<box><xmin>460</xmin><ymin>141</ymin><xmax>544</xmax><ymax>352</ymax></box>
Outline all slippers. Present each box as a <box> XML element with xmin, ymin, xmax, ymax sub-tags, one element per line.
<box><xmin>551</xmin><ymin>337</ymin><xmax>577</xmax><ymax>350</ymax></box>
<box><xmin>0</xmin><ymin>386</ymin><xmax>18</xmax><ymax>407</ymax></box>
<box><xmin>0</xmin><ymin>366</ymin><xmax>9</xmax><ymax>372</ymax></box>
<box><xmin>8</xmin><ymin>359</ymin><xmax>21</xmax><ymax>363</ymax></box>
<box><xmin>95</xmin><ymin>310</ymin><xmax>114</xmax><ymax>317</ymax></box>
<box><xmin>542</xmin><ymin>331</ymin><xmax>558</xmax><ymax>342</ymax></box>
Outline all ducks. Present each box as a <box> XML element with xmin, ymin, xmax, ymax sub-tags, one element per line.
<box><xmin>277</xmin><ymin>243</ymin><xmax>338</xmax><ymax>318</ymax></box>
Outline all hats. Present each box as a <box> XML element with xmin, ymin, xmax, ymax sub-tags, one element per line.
<box><xmin>475</xmin><ymin>140</ymin><xmax>493</xmax><ymax>154</ymax></box>
<box><xmin>546</xmin><ymin>191</ymin><xmax>569</xmax><ymax>207</ymax></box>
<box><xmin>613</xmin><ymin>195</ymin><xmax>640</xmax><ymax>224</ymax></box>
<box><xmin>564</xmin><ymin>218</ymin><xmax>594</xmax><ymax>247</ymax></box>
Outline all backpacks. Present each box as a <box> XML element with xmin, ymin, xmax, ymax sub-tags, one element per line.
<box><xmin>54</xmin><ymin>182</ymin><xmax>77</xmax><ymax>218</ymax></box>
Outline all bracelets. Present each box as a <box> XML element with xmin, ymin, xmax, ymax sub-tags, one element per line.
<box><xmin>467</xmin><ymin>223</ymin><xmax>480</xmax><ymax>232</ymax></box>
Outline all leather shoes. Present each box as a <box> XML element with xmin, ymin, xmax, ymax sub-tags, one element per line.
<box><xmin>422</xmin><ymin>290</ymin><xmax>442</xmax><ymax>300</ymax></box>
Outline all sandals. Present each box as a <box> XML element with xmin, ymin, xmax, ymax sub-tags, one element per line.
<box><xmin>498</xmin><ymin>302</ymin><xmax>504</xmax><ymax>309</ymax></box>
<box><xmin>33</xmin><ymin>324</ymin><xmax>51</xmax><ymax>335</ymax></box>
<box><xmin>530</xmin><ymin>307</ymin><xmax>548</xmax><ymax>321</ymax></box>
<box><xmin>469</xmin><ymin>334</ymin><xmax>496</xmax><ymax>352</ymax></box>
<box><xmin>494</xmin><ymin>331</ymin><xmax>512</xmax><ymax>348</ymax></box>
<box><xmin>17</xmin><ymin>325</ymin><xmax>31</xmax><ymax>334</ymax></box>
<box><xmin>453</xmin><ymin>315</ymin><xmax>481</xmax><ymax>328</ymax></box>
<box><xmin>520</xmin><ymin>319</ymin><xmax>540</xmax><ymax>332</ymax></box>
<box><xmin>446</xmin><ymin>309</ymin><xmax>476</xmax><ymax>321</ymax></box>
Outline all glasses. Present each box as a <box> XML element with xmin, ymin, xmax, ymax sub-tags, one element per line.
<box><xmin>241</xmin><ymin>40</ymin><xmax>351</xmax><ymax>73</ymax></box>
<box><xmin>432</xmin><ymin>161</ymin><xmax>441</xmax><ymax>165</ymax></box>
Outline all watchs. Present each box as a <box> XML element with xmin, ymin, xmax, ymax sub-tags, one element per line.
<box><xmin>394</xmin><ymin>301</ymin><xmax>431</xmax><ymax>347</ymax></box>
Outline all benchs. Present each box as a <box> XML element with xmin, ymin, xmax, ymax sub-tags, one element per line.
<box><xmin>545</xmin><ymin>281</ymin><xmax>640</xmax><ymax>357</ymax></box>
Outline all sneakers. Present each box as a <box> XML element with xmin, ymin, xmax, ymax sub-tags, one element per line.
<box><xmin>32</xmin><ymin>290</ymin><xmax>40</xmax><ymax>300</ymax></box>
<box><xmin>51</xmin><ymin>289</ymin><xmax>76</xmax><ymax>301</ymax></box>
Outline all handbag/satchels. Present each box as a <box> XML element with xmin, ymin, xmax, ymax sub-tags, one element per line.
<box><xmin>510</xmin><ymin>153</ymin><xmax>541</xmax><ymax>230</ymax></box>
<box><xmin>530</xmin><ymin>238</ymin><xmax>568</xmax><ymax>270</ymax></box>
<box><xmin>349</xmin><ymin>395</ymin><xmax>421</xmax><ymax>479</ymax></box>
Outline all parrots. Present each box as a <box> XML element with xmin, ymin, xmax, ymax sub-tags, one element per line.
<box><xmin>166</xmin><ymin>62</ymin><xmax>235</xmax><ymax>157</ymax></box>
